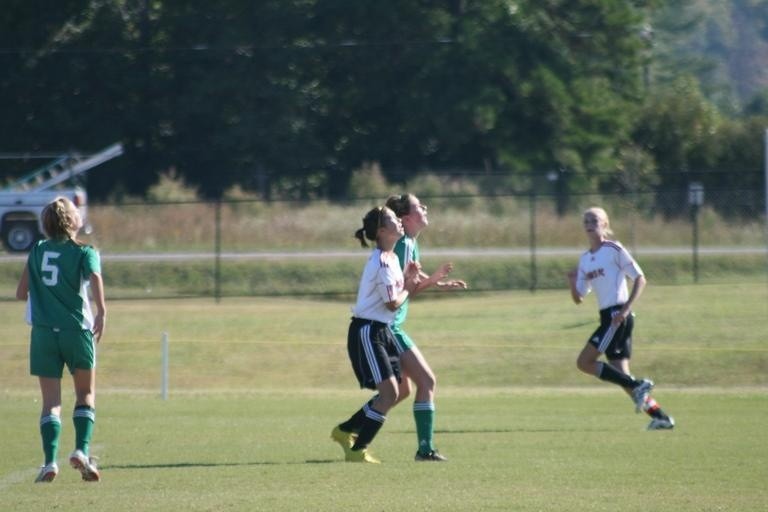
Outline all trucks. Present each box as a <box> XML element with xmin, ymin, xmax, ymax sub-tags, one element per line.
<box><xmin>1</xmin><ymin>187</ymin><xmax>94</xmax><ymax>251</ymax></box>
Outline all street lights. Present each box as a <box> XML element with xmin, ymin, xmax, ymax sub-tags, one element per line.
<box><xmin>687</xmin><ymin>181</ymin><xmax>708</xmax><ymax>287</ymax></box>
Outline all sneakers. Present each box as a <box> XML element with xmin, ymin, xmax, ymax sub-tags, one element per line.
<box><xmin>345</xmin><ymin>447</ymin><xmax>381</xmax><ymax>464</ymax></box>
<box><xmin>647</xmin><ymin>416</ymin><xmax>675</xmax><ymax>430</ymax></box>
<box><xmin>330</xmin><ymin>424</ymin><xmax>359</xmax><ymax>454</ymax></box>
<box><xmin>69</xmin><ymin>449</ymin><xmax>100</xmax><ymax>482</ymax></box>
<box><xmin>631</xmin><ymin>378</ymin><xmax>654</xmax><ymax>413</ymax></box>
<box><xmin>34</xmin><ymin>462</ymin><xmax>57</xmax><ymax>483</ymax></box>
<box><xmin>415</xmin><ymin>448</ymin><xmax>446</xmax><ymax>461</ymax></box>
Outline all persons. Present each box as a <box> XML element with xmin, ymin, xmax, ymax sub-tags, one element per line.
<box><xmin>330</xmin><ymin>205</ymin><xmax>421</xmax><ymax>464</ymax></box>
<box><xmin>568</xmin><ymin>206</ymin><xmax>675</xmax><ymax>431</ymax></box>
<box><xmin>14</xmin><ymin>195</ymin><xmax>106</xmax><ymax>483</ymax></box>
<box><xmin>387</xmin><ymin>191</ymin><xmax>467</xmax><ymax>460</ymax></box>
